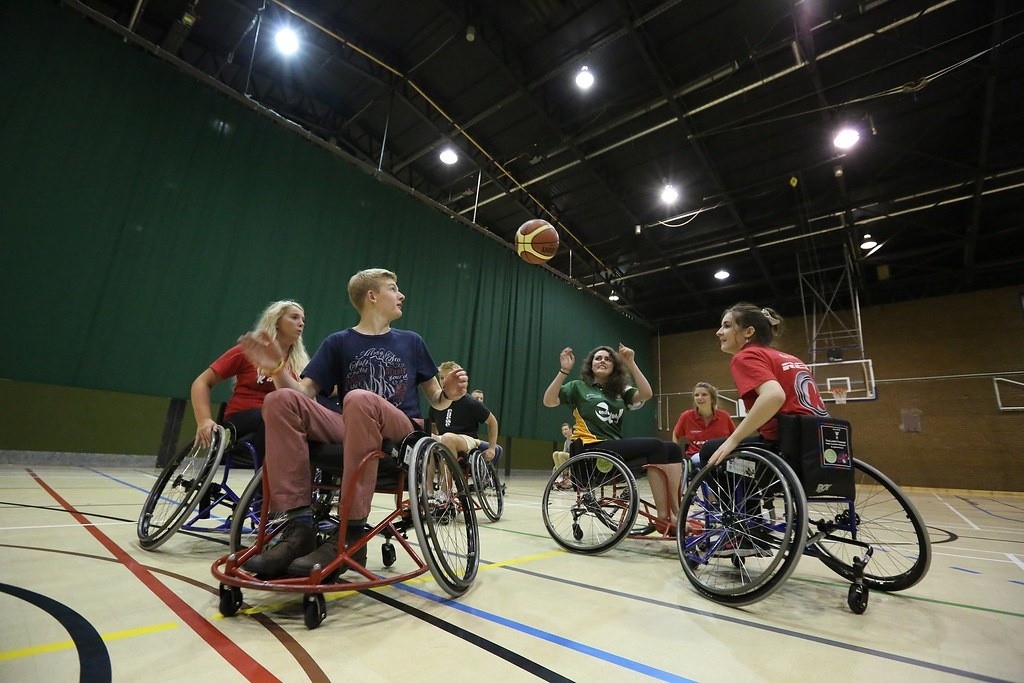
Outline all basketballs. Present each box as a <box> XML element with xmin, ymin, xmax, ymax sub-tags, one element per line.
<box><xmin>514</xmin><ymin>219</ymin><xmax>560</xmax><ymax>267</ymax></box>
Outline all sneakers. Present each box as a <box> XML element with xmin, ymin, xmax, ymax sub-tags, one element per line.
<box><xmin>289</xmin><ymin>524</ymin><xmax>367</xmax><ymax>577</ymax></box>
<box><xmin>242</xmin><ymin>518</ymin><xmax>322</xmax><ymax>573</ymax></box>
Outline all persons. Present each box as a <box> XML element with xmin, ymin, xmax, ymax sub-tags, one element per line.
<box><xmin>191</xmin><ymin>299</ymin><xmax>344</xmax><ymax>534</ymax></box>
<box><xmin>552</xmin><ymin>422</ymin><xmax>574</xmax><ymax>489</ymax></box>
<box><xmin>542</xmin><ymin>341</ymin><xmax>706</xmax><ymax>536</ymax></box>
<box><xmin>399</xmin><ymin>360</ymin><xmax>504</xmax><ymax>527</ymax></box>
<box><xmin>242</xmin><ymin>266</ymin><xmax>470</xmax><ymax>575</ymax></box>
<box><xmin>671</xmin><ymin>381</ymin><xmax>737</xmax><ymax>501</ymax></box>
<box><xmin>695</xmin><ymin>303</ymin><xmax>834</xmax><ymax>559</ymax></box>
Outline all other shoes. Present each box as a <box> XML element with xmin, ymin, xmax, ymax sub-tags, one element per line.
<box><xmin>316</xmin><ymin>519</ymin><xmax>338</xmax><ymax>536</ymax></box>
<box><xmin>752</xmin><ymin>536</ymin><xmax>774</xmax><ymax>557</ymax></box>
<box><xmin>432</xmin><ymin>498</ymin><xmax>451</xmax><ymax>525</ymax></box>
<box><xmin>655</xmin><ymin>518</ymin><xmax>706</xmax><ymax>537</ymax></box>
<box><xmin>708</xmin><ymin>499</ymin><xmax>720</xmax><ymax>513</ymax></box>
<box><xmin>402</xmin><ymin>507</ymin><xmax>425</xmax><ymax>516</ymax></box>
<box><xmin>704</xmin><ymin>534</ymin><xmax>762</xmax><ymax>557</ymax></box>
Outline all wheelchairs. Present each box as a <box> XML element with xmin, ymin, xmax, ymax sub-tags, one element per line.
<box><xmin>211</xmin><ymin>416</ymin><xmax>480</xmax><ymax>630</ymax></box>
<box><xmin>541</xmin><ymin>438</ymin><xmax>704</xmax><ymax>555</ymax></box>
<box><xmin>675</xmin><ymin>412</ymin><xmax>932</xmax><ymax>615</ymax></box>
<box><xmin>417</xmin><ymin>447</ymin><xmax>507</xmax><ymax>522</ymax></box>
<box><xmin>137</xmin><ymin>400</ymin><xmax>341</xmax><ymax>552</ymax></box>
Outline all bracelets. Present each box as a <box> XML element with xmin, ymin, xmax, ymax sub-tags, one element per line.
<box><xmin>439</xmin><ymin>386</ymin><xmax>450</xmax><ymax>403</ymax></box>
<box><xmin>559</xmin><ymin>369</ymin><xmax>568</xmax><ymax>375</ymax></box>
<box><xmin>266</xmin><ymin>358</ymin><xmax>285</xmax><ymax>375</ymax></box>
